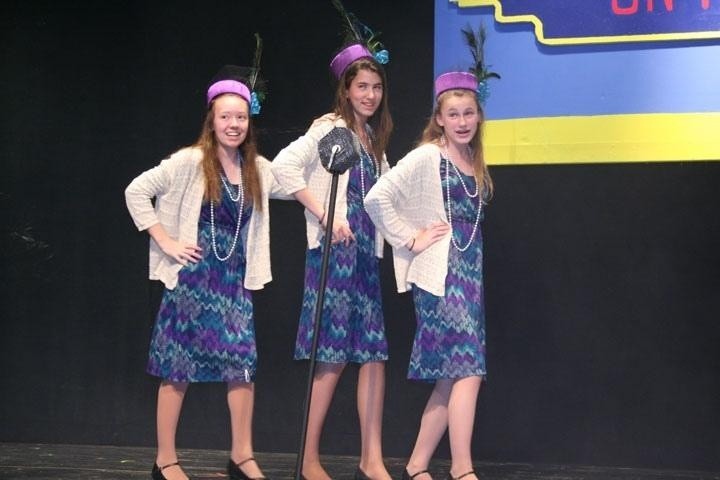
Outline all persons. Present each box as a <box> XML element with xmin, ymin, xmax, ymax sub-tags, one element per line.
<box><xmin>124</xmin><ymin>75</ymin><xmax>296</xmax><ymax>480</ymax></box>
<box><xmin>270</xmin><ymin>42</ymin><xmax>393</xmax><ymax>480</ymax></box>
<box><xmin>362</xmin><ymin>72</ymin><xmax>493</xmax><ymax>480</ymax></box>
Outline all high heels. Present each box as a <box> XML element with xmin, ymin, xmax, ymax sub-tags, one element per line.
<box><xmin>446</xmin><ymin>470</ymin><xmax>474</xmax><ymax>479</ymax></box>
<box><xmin>401</xmin><ymin>466</ymin><xmax>429</xmax><ymax>479</ymax></box>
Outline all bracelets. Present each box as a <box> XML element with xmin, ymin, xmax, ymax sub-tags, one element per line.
<box><xmin>408</xmin><ymin>231</ymin><xmax>418</xmax><ymax>253</ymax></box>
<box><xmin>319</xmin><ymin>207</ymin><xmax>326</xmax><ymax>224</ymax></box>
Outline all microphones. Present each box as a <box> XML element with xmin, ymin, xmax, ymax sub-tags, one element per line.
<box><xmin>317</xmin><ymin>128</ymin><xmax>359</xmax><ymax>174</ymax></box>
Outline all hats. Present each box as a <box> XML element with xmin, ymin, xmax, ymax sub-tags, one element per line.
<box><xmin>207</xmin><ymin>80</ymin><xmax>251</xmax><ymax>108</ymax></box>
<box><xmin>436</xmin><ymin>72</ymin><xmax>479</xmax><ymax>99</ymax></box>
<box><xmin>329</xmin><ymin>43</ymin><xmax>373</xmax><ymax>79</ymax></box>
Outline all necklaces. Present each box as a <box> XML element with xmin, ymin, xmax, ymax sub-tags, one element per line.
<box><xmin>210</xmin><ymin>149</ymin><xmax>245</xmax><ymax>262</ymax></box>
<box><xmin>440</xmin><ymin>134</ymin><xmax>482</xmax><ymax>252</ymax></box>
<box><xmin>350</xmin><ymin>129</ymin><xmax>381</xmax><ymax>215</ymax></box>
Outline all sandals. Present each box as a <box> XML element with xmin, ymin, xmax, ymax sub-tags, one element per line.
<box><xmin>354</xmin><ymin>466</ymin><xmax>373</xmax><ymax>480</ymax></box>
<box><xmin>228</xmin><ymin>458</ymin><xmax>268</xmax><ymax>480</ymax></box>
<box><xmin>151</xmin><ymin>462</ymin><xmax>191</xmax><ymax>480</ymax></box>
